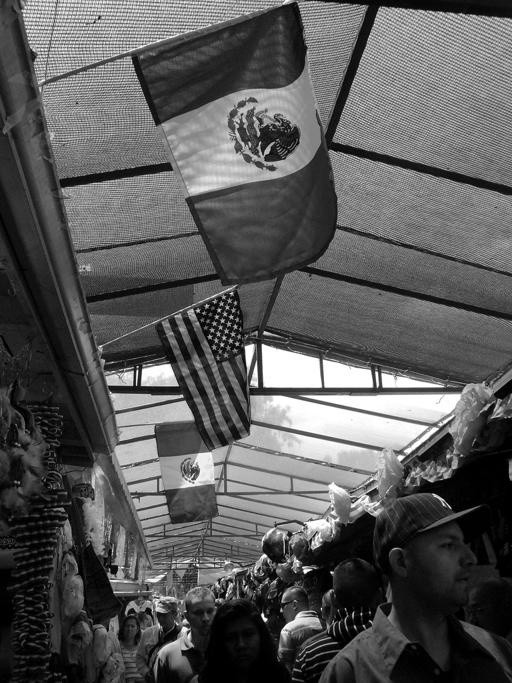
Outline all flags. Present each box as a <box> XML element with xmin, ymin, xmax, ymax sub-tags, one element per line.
<box><xmin>153</xmin><ymin>420</ymin><xmax>218</xmax><ymax>525</ymax></box>
<box><xmin>127</xmin><ymin>0</ymin><xmax>340</xmax><ymax>284</ymax></box>
<box><xmin>155</xmin><ymin>288</ymin><xmax>252</xmax><ymax>448</ymax></box>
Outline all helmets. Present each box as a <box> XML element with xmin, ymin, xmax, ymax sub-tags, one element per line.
<box><xmin>262</xmin><ymin>528</ymin><xmax>287</xmax><ymax>561</ymax></box>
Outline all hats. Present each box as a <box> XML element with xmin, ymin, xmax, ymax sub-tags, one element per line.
<box><xmin>154</xmin><ymin>596</ymin><xmax>177</xmax><ymax>615</ymax></box>
<box><xmin>373</xmin><ymin>493</ymin><xmax>480</xmax><ymax>561</ymax></box>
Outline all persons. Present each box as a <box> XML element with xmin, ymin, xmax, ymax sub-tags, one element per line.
<box><xmin>463</xmin><ymin>577</ymin><xmax>510</xmax><ymax>640</ymax></box>
<box><xmin>320</xmin><ymin>589</ymin><xmax>343</xmax><ymax>630</ymax></box>
<box><xmin>318</xmin><ymin>492</ymin><xmax>509</xmax><ymax>681</ymax></box>
<box><xmin>292</xmin><ymin>558</ymin><xmax>383</xmax><ymax>682</ymax></box>
<box><xmin>1</xmin><ymin>492</ymin><xmax>292</xmax><ymax>681</ymax></box>
<box><xmin>276</xmin><ymin>585</ymin><xmax>323</xmax><ymax>678</ymax></box>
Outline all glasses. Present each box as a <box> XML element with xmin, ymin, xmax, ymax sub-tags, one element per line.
<box><xmin>280</xmin><ymin>600</ymin><xmax>294</xmax><ymax>609</ymax></box>
